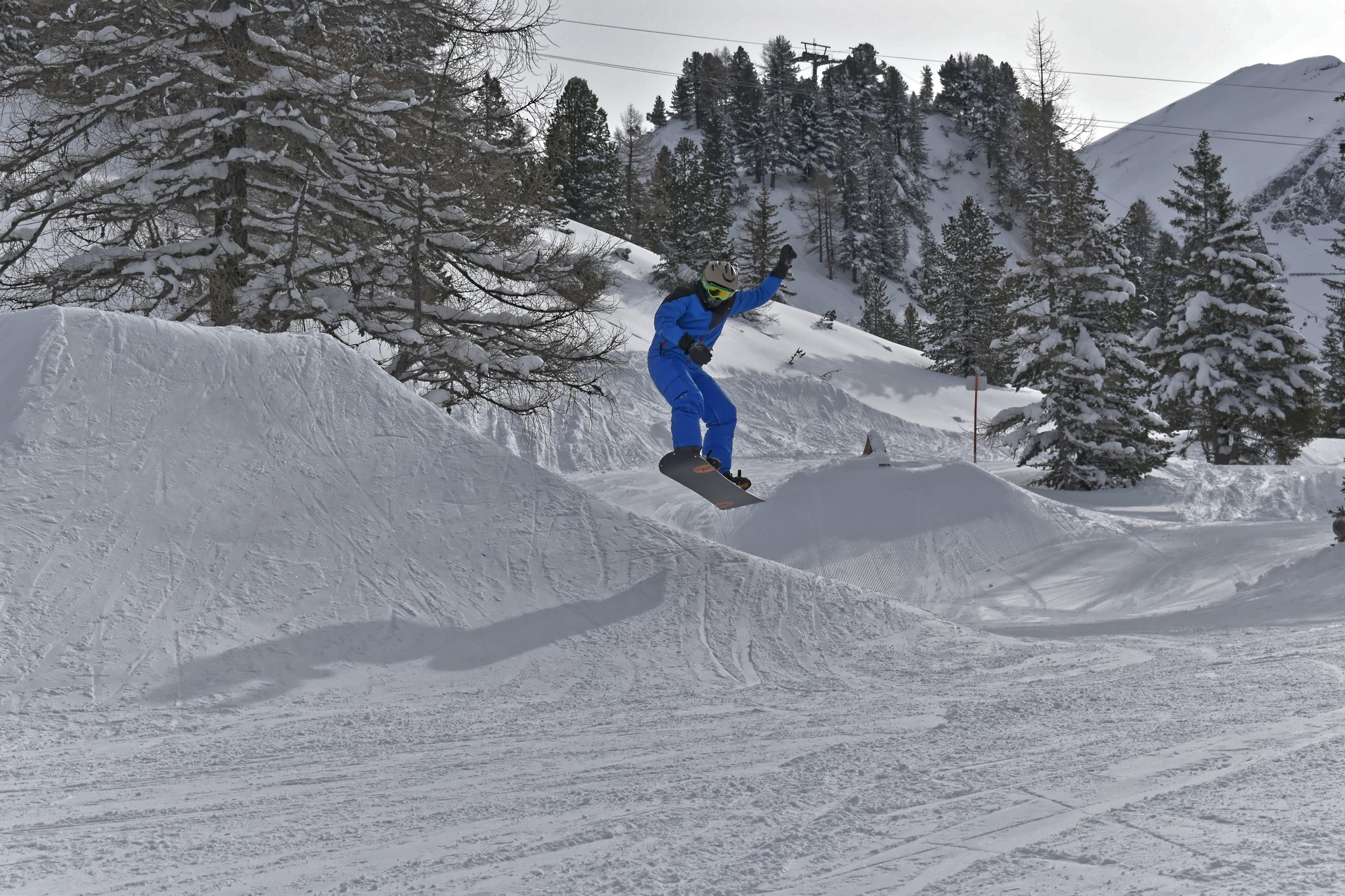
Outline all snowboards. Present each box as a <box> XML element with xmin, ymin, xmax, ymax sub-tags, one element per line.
<box><xmin>657</xmin><ymin>450</ymin><xmax>765</xmax><ymax>511</ymax></box>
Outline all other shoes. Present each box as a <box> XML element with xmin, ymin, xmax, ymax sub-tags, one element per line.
<box><xmin>723</xmin><ymin>471</ymin><xmax>752</xmax><ymax>491</ymax></box>
<box><xmin>704</xmin><ymin>457</ymin><xmax>722</xmax><ymax>472</ymax></box>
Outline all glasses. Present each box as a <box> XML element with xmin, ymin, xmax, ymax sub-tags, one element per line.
<box><xmin>707</xmin><ymin>283</ymin><xmax>733</xmax><ymax>301</ymax></box>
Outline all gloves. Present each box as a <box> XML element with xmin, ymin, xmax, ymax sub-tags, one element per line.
<box><xmin>678</xmin><ymin>332</ymin><xmax>713</xmax><ymax>366</ymax></box>
<box><xmin>770</xmin><ymin>244</ymin><xmax>797</xmax><ymax>279</ymax></box>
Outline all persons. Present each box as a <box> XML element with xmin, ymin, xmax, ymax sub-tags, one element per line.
<box><xmin>647</xmin><ymin>244</ymin><xmax>797</xmax><ymax>492</ymax></box>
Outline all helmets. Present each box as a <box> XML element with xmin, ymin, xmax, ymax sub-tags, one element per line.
<box><xmin>704</xmin><ymin>261</ymin><xmax>739</xmax><ymax>292</ymax></box>
